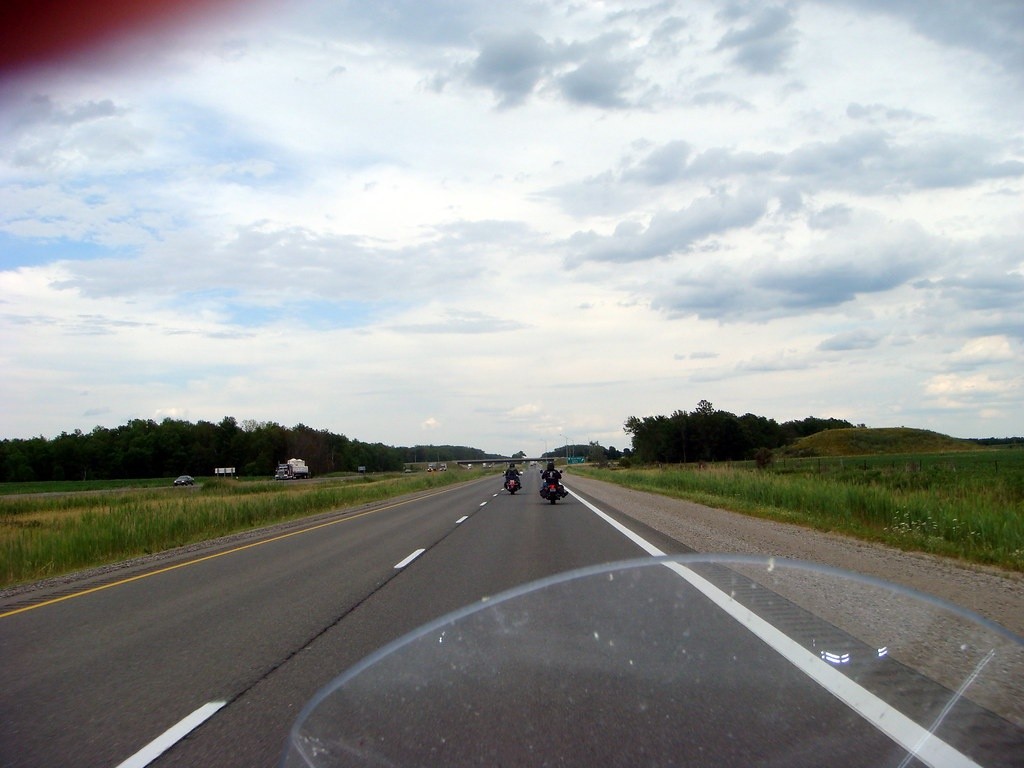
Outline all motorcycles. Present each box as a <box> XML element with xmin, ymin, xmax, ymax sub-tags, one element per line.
<box><xmin>503</xmin><ymin>470</ymin><xmax>523</xmax><ymax>495</ymax></box>
<box><xmin>540</xmin><ymin>469</ymin><xmax>563</xmax><ymax>504</ymax></box>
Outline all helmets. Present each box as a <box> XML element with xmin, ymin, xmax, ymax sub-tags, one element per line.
<box><xmin>510</xmin><ymin>464</ymin><xmax>515</xmax><ymax>467</ymax></box>
<box><xmin>547</xmin><ymin>462</ymin><xmax>554</xmax><ymax>469</ymax></box>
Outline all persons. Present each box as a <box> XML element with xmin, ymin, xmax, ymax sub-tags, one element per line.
<box><xmin>504</xmin><ymin>464</ymin><xmax>522</xmax><ymax>489</ymax></box>
<box><xmin>540</xmin><ymin>462</ymin><xmax>568</xmax><ymax>498</ymax></box>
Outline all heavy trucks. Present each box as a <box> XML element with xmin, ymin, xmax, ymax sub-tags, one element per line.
<box><xmin>483</xmin><ymin>463</ymin><xmax>489</xmax><ymax>468</ymax></box>
<box><xmin>274</xmin><ymin>464</ymin><xmax>311</xmax><ymax>480</ymax></box>
<box><xmin>468</xmin><ymin>464</ymin><xmax>473</xmax><ymax>469</ymax></box>
<box><xmin>440</xmin><ymin>463</ymin><xmax>446</xmax><ymax>471</ymax></box>
<box><xmin>428</xmin><ymin>465</ymin><xmax>436</xmax><ymax>471</ymax></box>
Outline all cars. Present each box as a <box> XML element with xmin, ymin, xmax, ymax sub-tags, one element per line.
<box><xmin>174</xmin><ymin>476</ymin><xmax>195</xmax><ymax>487</ymax></box>
<box><xmin>405</xmin><ymin>468</ymin><xmax>411</xmax><ymax>473</ymax></box>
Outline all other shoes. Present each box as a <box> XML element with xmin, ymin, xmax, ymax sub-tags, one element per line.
<box><xmin>519</xmin><ymin>487</ymin><xmax>522</xmax><ymax>489</ymax></box>
<box><xmin>562</xmin><ymin>491</ymin><xmax>568</xmax><ymax>498</ymax></box>
